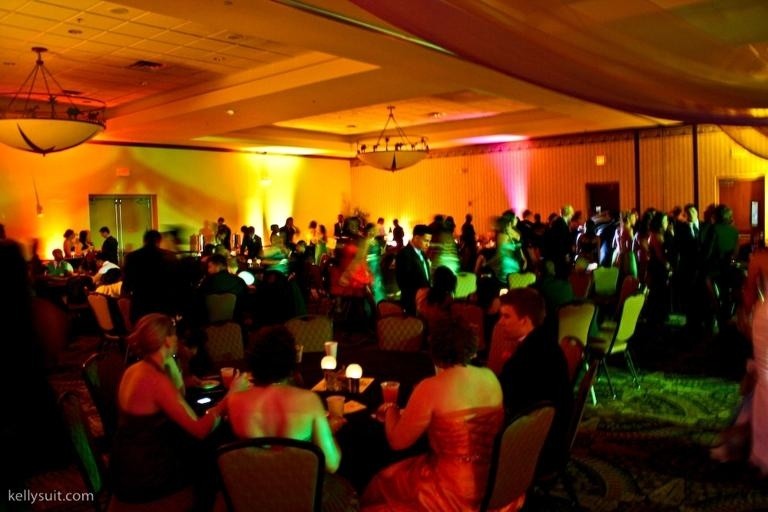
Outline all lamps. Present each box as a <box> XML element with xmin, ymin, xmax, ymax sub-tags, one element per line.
<box><xmin>0</xmin><ymin>47</ymin><xmax>107</xmax><ymax>156</ymax></box>
<box><xmin>356</xmin><ymin>104</ymin><xmax>430</xmax><ymax>172</ymax></box>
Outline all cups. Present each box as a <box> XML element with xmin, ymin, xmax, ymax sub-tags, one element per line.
<box><xmin>324</xmin><ymin>341</ymin><xmax>338</xmax><ymax>359</ymax></box>
<box><xmin>326</xmin><ymin>395</ymin><xmax>346</xmax><ymax>420</ymax></box>
<box><xmin>345</xmin><ymin>378</ymin><xmax>359</xmax><ymax>395</ymax></box>
<box><xmin>296</xmin><ymin>344</ymin><xmax>303</xmax><ymax>363</ymax></box>
<box><xmin>323</xmin><ymin>369</ymin><xmax>336</xmax><ymax>390</ymax></box>
<box><xmin>381</xmin><ymin>380</ymin><xmax>400</xmax><ymax>405</ymax></box>
<box><xmin>221</xmin><ymin>367</ymin><xmax>234</xmax><ymax>388</ymax></box>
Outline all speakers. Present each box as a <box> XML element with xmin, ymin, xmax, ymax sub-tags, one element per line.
<box><xmin>585</xmin><ymin>181</ymin><xmax>619</xmax><ymax>220</ymax></box>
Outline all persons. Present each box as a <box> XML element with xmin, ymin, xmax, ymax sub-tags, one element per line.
<box><xmin>0</xmin><ymin>203</ymin><xmax>768</xmax><ymax>510</ymax></box>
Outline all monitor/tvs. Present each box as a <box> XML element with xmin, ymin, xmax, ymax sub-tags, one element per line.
<box><xmin>750</xmin><ymin>200</ymin><xmax>760</xmax><ymax>227</ymax></box>
